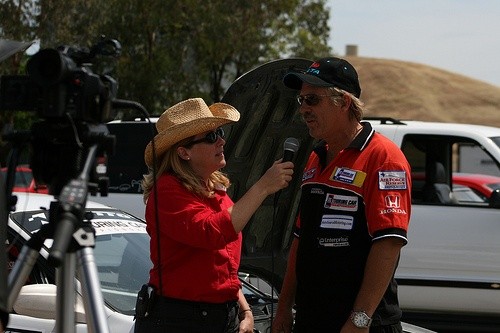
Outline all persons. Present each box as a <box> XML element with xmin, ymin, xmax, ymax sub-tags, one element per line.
<box><xmin>141</xmin><ymin>97</ymin><xmax>293</xmax><ymax>333</ymax></box>
<box><xmin>272</xmin><ymin>58</ymin><xmax>412</xmax><ymax>333</ymax></box>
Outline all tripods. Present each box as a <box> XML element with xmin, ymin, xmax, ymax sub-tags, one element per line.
<box><xmin>0</xmin><ymin>133</ymin><xmax>111</xmax><ymax>333</ymax></box>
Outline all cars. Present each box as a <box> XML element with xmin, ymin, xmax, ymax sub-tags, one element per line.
<box><xmin>408</xmin><ymin>170</ymin><xmax>500</xmax><ymax>203</ymax></box>
<box><xmin>360</xmin><ymin>115</ymin><xmax>499</xmax><ymax>320</ymax></box>
<box><xmin>0</xmin><ymin>57</ymin><xmax>436</xmax><ymax>333</ymax></box>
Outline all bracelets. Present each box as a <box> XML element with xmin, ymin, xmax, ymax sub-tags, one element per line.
<box><xmin>239</xmin><ymin>309</ymin><xmax>252</xmax><ymax>315</ymax></box>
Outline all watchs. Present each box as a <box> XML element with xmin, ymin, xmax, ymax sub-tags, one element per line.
<box><xmin>349</xmin><ymin>310</ymin><xmax>372</xmax><ymax>328</ymax></box>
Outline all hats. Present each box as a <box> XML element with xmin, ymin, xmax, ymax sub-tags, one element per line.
<box><xmin>145</xmin><ymin>98</ymin><xmax>240</xmax><ymax>169</ymax></box>
<box><xmin>283</xmin><ymin>57</ymin><xmax>361</xmax><ymax>99</ymax></box>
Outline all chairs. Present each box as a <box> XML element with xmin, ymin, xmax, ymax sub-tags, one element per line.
<box><xmin>422</xmin><ymin>161</ymin><xmax>458</xmax><ymax>204</ymax></box>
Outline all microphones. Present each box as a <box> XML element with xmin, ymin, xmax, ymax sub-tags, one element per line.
<box><xmin>272</xmin><ymin>137</ymin><xmax>300</xmax><ymax>209</ymax></box>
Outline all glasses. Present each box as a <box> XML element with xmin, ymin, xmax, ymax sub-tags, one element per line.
<box><xmin>183</xmin><ymin>128</ymin><xmax>225</xmax><ymax>147</ymax></box>
<box><xmin>297</xmin><ymin>93</ymin><xmax>344</xmax><ymax>106</ymax></box>
